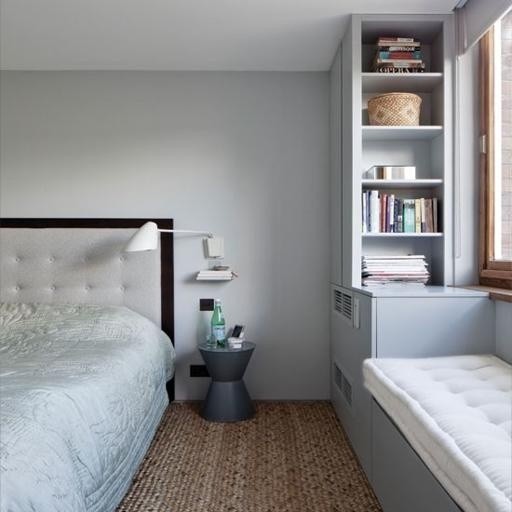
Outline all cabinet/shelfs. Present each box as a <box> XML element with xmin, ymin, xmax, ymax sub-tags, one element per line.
<box><xmin>211</xmin><ymin>299</ymin><xmax>225</xmax><ymax>347</ymax></box>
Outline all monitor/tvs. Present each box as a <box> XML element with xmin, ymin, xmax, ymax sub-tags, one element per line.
<box><xmin>361</xmin><ymin>254</ymin><xmax>431</xmax><ymax>287</ymax></box>
<box><xmin>362</xmin><ymin>189</ymin><xmax>438</xmax><ymax>233</ymax></box>
<box><xmin>370</xmin><ymin>37</ymin><xmax>425</xmax><ymax>73</ymax></box>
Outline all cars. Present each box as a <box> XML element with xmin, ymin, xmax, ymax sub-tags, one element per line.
<box><xmin>206</xmin><ymin>335</ymin><xmax>217</xmax><ymax>348</ymax></box>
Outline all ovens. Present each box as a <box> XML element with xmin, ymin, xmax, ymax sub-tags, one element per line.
<box><xmin>0</xmin><ymin>218</ymin><xmax>176</xmax><ymax>512</ymax></box>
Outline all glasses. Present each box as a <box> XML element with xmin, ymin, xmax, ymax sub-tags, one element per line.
<box><xmin>125</xmin><ymin>222</ymin><xmax>224</xmax><ymax>258</ymax></box>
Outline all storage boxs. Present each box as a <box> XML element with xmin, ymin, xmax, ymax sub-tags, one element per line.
<box><xmin>363</xmin><ymin>354</ymin><xmax>512</xmax><ymax>512</ymax></box>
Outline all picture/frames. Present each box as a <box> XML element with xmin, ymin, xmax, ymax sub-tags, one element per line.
<box><xmin>198</xmin><ymin>341</ymin><xmax>256</xmax><ymax>422</ymax></box>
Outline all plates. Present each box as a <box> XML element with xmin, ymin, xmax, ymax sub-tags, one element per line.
<box><xmin>328</xmin><ymin>14</ymin><xmax>494</xmax><ymax>485</ymax></box>
